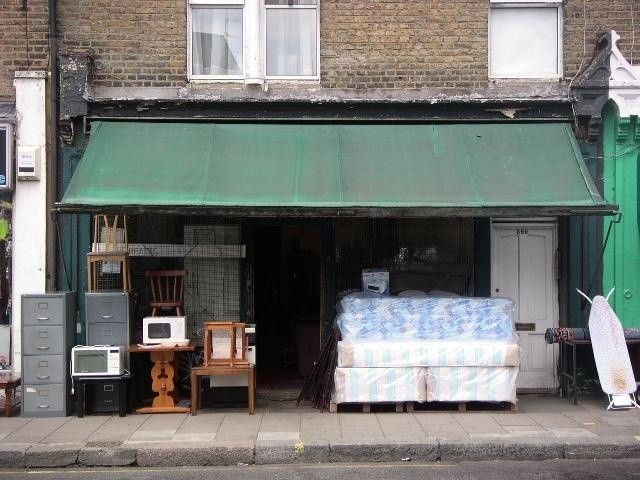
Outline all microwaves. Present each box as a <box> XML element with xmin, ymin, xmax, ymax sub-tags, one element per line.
<box><xmin>71</xmin><ymin>344</ymin><xmax>124</xmax><ymax>377</ymax></box>
<box><xmin>143</xmin><ymin>316</ymin><xmax>187</xmax><ymax>345</ymax></box>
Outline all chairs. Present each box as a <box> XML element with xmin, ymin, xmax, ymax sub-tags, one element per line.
<box><xmin>87</xmin><ymin>213</ymin><xmax>132</xmax><ymax>292</ymax></box>
<box><xmin>144</xmin><ymin>270</ymin><xmax>190</xmax><ymax>317</ymax></box>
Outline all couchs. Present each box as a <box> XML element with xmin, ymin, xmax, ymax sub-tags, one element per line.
<box><xmin>327</xmin><ymin>293</ymin><xmax>521</xmax><ymax>412</ymax></box>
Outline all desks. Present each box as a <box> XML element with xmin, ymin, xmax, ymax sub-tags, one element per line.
<box><xmin>0</xmin><ymin>369</ymin><xmax>21</xmax><ymax>416</ymax></box>
<box><xmin>559</xmin><ymin>337</ymin><xmax>640</xmax><ymax>405</ymax></box>
<box><xmin>128</xmin><ymin>338</ymin><xmax>198</xmax><ymax>414</ymax></box>
<box><xmin>190</xmin><ymin>362</ymin><xmax>256</xmax><ymax>417</ymax></box>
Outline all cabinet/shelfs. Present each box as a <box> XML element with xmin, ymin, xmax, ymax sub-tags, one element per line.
<box><xmin>21</xmin><ymin>290</ymin><xmax>75</xmax><ymax>418</ymax></box>
<box><xmin>84</xmin><ymin>291</ymin><xmax>131</xmax><ymax>370</ymax></box>
